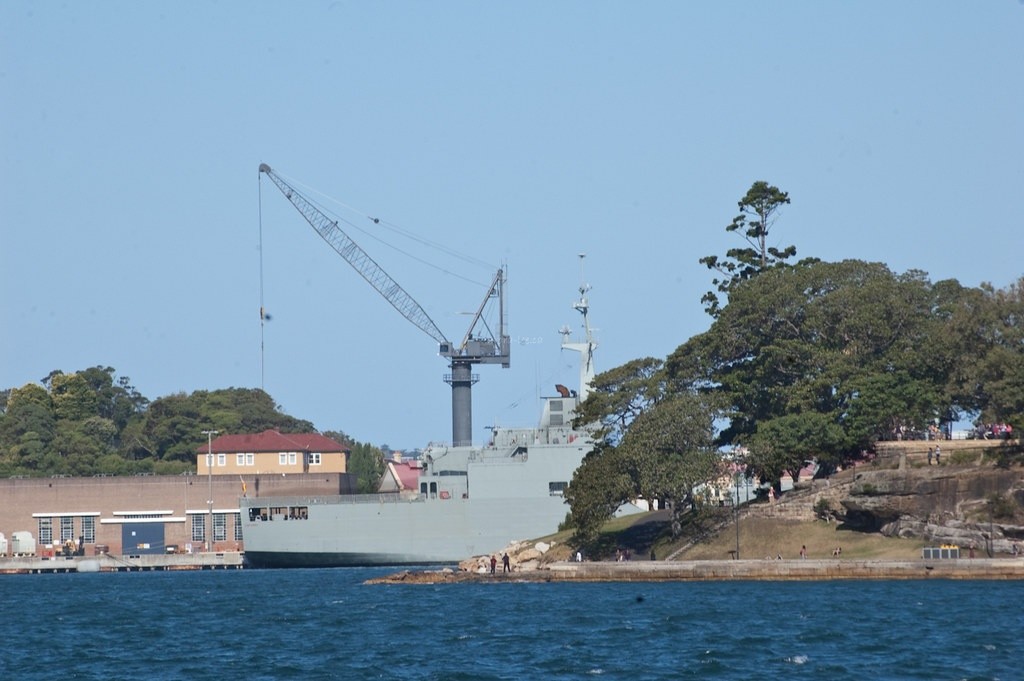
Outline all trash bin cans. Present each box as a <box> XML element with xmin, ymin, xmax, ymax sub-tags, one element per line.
<box><xmin>94</xmin><ymin>545</ymin><xmax>109</xmax><ymax>556</ymax></box>
<box><xmin>11</xmin><ymin>531</ymin><xmax>36</xmax><ymax>557</ymax></box>
<box><xmin>0</xmin><ymin>533</ymin><xmax>8</xmax><ymax>555</ymax></box>
<box><xmin>166</xmin><ymin>545</ymin><xmax>177</xmax><ymax>553</ymax></box>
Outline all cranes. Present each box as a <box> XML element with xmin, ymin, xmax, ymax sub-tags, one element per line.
<box><xmin>258</xmin><ymin>164</ymin><xmax>510</xmax><ymax>446</ymax></box>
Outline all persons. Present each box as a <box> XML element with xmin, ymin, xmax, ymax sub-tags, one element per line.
<box><xmin>777</xmin><ymin>554</ymin><xmax>782</xmax><ymax>560</ymax></box>
<box><xmin>832</xmin><ymin>546</ymin><xmax>841</xmax><ymax>556</ymax></box>
<box><xmin>800</xmin><ymin>545</ymin><xmax>807</xmax><ymax>559</ymax></box>
<box><xmin>768</xmin><ymin>487</ymin><xmax>774</xmax><ymax>502</ymax></box>
<box><xmin>893</xmin><ymin>421</ymin><xmax>1013</xmax><ymax>466</ymax></box>
<box><xmin>615</xmin><ymin>549</ymin><xmax>630</xmax><ymax>562</ymax></box>
<box><xmin>1013</xmin><ymin>540</ymin><xmax>1019</xmax><ymax>557</ymax></box>
<box><xmin>576</xmin><ymin>551</ymin><xmax>582</xmax><ymax>562</ymax></box>
<box><xmin>490</xmin><ymin>555</ymin><xmax>497</xmax><ymax>573</ymax></box>
<box><xmin>502</xmin><ymin>553</ymin><xmax>511</xmax><ymax>573</ymax></box>
<box><xmin>810</xmin><ymin>459</ymin><xmax>852</xmax><ymax>490</ymax></box>
<box><xmin>969</xmin><ymin>545</ymin><xmax>975</xmax><ymax>558</ymax></box>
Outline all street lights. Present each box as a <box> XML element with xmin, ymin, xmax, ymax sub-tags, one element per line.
<box><xmin>201</xmin><ymin>430</ymin><xmax>219</xmax><ymax>552</ymax></box>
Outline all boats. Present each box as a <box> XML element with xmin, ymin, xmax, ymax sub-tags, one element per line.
<box><xmin>240</xmin><ymin>256</ymin><xmax>658</xmax><ymax>567</ymax></box>
